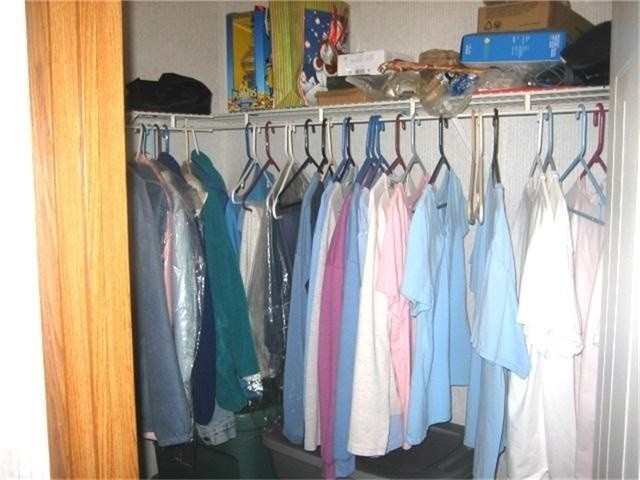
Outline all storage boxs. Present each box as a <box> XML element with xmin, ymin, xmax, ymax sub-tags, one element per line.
<box><xmin>477</xmin><ymin>1</ymin><xmax>595</xmax><ymax>45</ymax></box>
<box><xmin>262</xmin><ymin>421</ymin><xmax>474</xmax><ymax>480</ymax></box>
<box><xmin>460</xmin><ymin>31</ymin><xmax>571</xmax><ymax>68</ymax></box>
<box><xmin>153</xmin><ymin>404</ymin><xmax>284</xmax><ymax>480</ymax></box>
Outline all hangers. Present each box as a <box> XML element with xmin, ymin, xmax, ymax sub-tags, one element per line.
<box><xmin>132</xmin><ymin>102</ymin><xmax>609</xmax><ymax>230</ymax></box>
